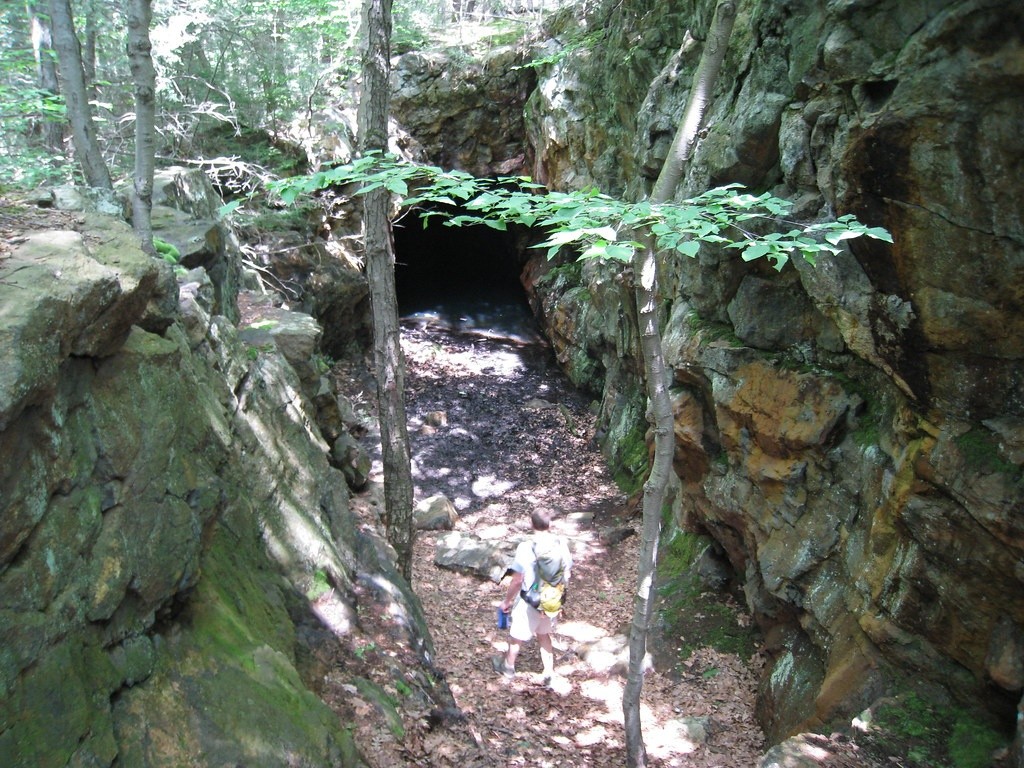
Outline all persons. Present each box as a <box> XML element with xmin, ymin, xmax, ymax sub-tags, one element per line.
<box><xmin>491</xmin><ymin>507</ymin><xmax>573</xmax><ymax>686</ymax></box>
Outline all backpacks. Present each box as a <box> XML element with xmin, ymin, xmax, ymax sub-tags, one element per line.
<box><xmin>522</xmin><ymin>538</ymin><xmax>568</xmax><ymax>621</ymax></box>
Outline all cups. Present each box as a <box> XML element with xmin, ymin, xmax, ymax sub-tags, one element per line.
<box><xmin>498</xmin><ymin>607</ymin><xmax>508</xmax><ymax>629</ymax></box>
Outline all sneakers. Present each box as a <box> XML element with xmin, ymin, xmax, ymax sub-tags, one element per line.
<box><xmin>540</xmin><ymin>674</ymin><xmax>553</xmax><ymax>686</ymax></box>
<box><xmin>492</xmin><ymin>655</ymin><xmax>515</xmax><ymax>679</ymax></box>
